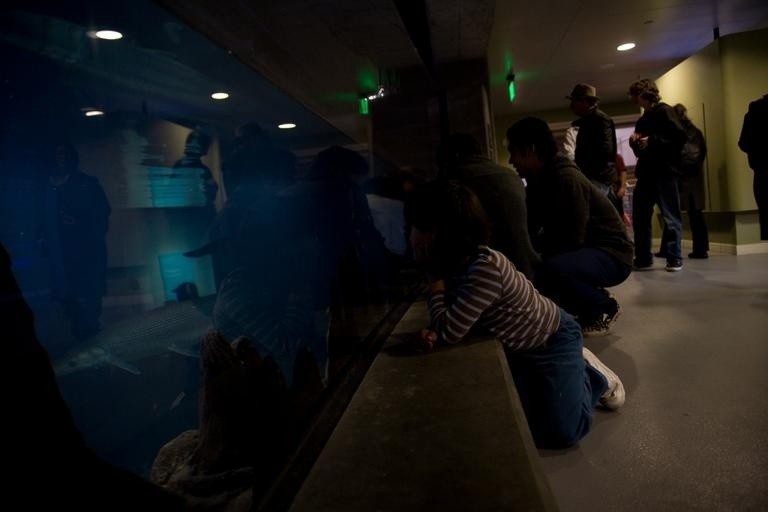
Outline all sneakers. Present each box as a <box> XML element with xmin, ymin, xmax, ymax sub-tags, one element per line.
<box><xmin>582</xmin><ymin>298</ymin><xmax>623</xmax><ymax>338</ymax></box>
<box><xmin>633</xmin><ymin>250</ymin><xmax>708</xmax><ymax>271</ymax></box>
<box><xmin>582</xmin><ymin>346</ymin><xmax>626</xmax><ymax>410</ymax></box>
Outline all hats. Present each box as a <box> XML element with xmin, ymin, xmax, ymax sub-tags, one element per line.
<box><xmin>565</xmin><ymin>84</ymin><xmax>600</xmax><ymax>100</ymax></box>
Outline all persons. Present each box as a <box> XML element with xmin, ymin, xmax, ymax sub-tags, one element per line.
<box><xmin>435</xmin><ymin>132</ymin><xmax>544</xmax><ymax>282</ymax></box>
<box><xmin>404</xmin><ymin>174</ymin><xmax>626</xmax><ymax>450</ymax></box>
<box><xmin>738</xmin><ymin>95</ymin><xmax>768</xmax><ymax>240</ymax></box>
<box><xmin>506</xmin><ymin>116</ymin><xmax>634</xmax><ymax>336</ymax></box>
<box><xmin>626</xmin><ymin>78</ymin><xmax>688</xmax><ymax>272</ymax></box>
<box><xmin>166</xmin><ymin>129</ymin><xmax>397</xmax><ymax>359</ymax></box>
<box><xmin>555</xmin><ymin>82</ymin><xmax>617</xmax><ymax>210</ymax></box>
<box><xmin>654</xmin><ymin>104</ymin><xmax>709</xmax><ymax>259</ymax></box>
<box><xmin>55</xmin><ymin>131</ymin><xmax>111</xmax><ymax>333</ymax></box>
<box><xmin>615</xmin><ymin>154</ymin><xmax>626</xmax><ymax>218</ymax></box>
